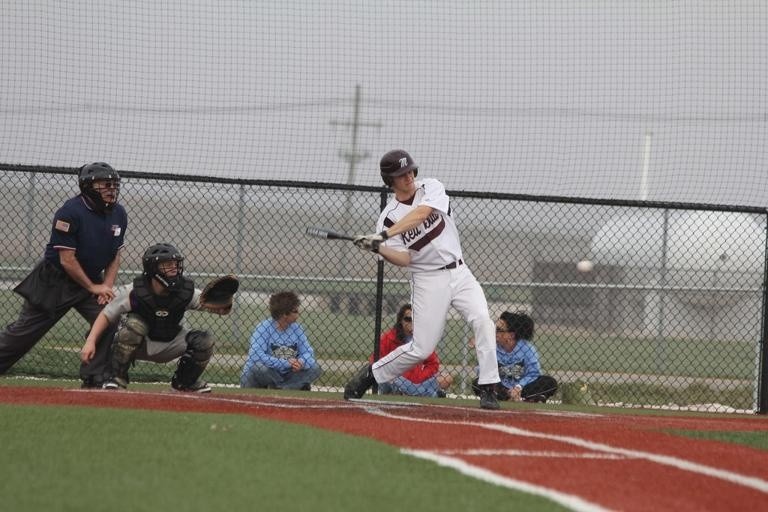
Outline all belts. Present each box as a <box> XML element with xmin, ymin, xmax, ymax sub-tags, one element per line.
<box><xmin>439</xmin><ymin>259</ymin><xmax>463</xmax><ymax>270</ymax></box>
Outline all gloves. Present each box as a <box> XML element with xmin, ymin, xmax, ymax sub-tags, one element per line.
<box><xmin>352</xmin><ymin>230</ymin><xmax>388</xmax><ymax>254</ymax></box>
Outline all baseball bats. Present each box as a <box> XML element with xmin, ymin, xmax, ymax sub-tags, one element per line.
<box><xmin>305</xmin><ymin>225</ymin><xmax>378</xmax><ymax>246</ymax></box>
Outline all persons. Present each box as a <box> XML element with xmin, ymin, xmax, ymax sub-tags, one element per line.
<box><xmin>0</xmin><ymin>160</ymin><xmax>128</xmax><ymax>392</ymax></box>
<box><xmin>467</xmin><ymin>310</ymin><xmax>558</xmax><ymax>406</ymax></box>
<box><xmin>79</xmin><ymin>241</ymin><xmax>240</xmax><ymax>394</ymax></box>
<box><xmin>239</xmin><ymin>289</ymin><xmax>321</xmax><ymax>391</ymax></box>
<box><xmin>365</xmin><ymin>301</ymin><xmax>452</xmax><ymax>399</ymax></box>
<box><xmin>342</xmin><ymin>147</ymin><xmax>502</xmax><ymax>411</ymax></box>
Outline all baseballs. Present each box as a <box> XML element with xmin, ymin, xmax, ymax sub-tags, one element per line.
<box><xmin>577</xmin><ymin>260</ymin><xmax>592</xmax><ymax>272</ymax></box>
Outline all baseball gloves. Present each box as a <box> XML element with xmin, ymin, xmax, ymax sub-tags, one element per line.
<box><xmin>200</xmin><ymin>273</ymin><xmax>239</xmax><ymax>315</ymax></box>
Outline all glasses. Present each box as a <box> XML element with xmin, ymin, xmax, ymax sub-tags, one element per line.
<box><xmin>403</xmin><ymin>316</ymin><xmax>412</xmax><ymax>322</ymax></box>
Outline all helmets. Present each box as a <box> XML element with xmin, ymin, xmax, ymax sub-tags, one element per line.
<box><xmin>141</xmin><ymin>243</ymin><xmax>184</xmax><ymax>292</ymax></box>
<box><xmin>79</xmin><ymin>162</ymin><xmax>120</xmax><ymax>210</ymax></box>
<box><xmin>380</xmin><ymin>149</ymin><xmax>419</xmax><ymax>186</ymax></box>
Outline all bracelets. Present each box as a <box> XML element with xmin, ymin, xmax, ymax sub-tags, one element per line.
<box><xmin>512</xmin><ymin>388</ymin><xmax>519</xmax><ymax>394</ymax></box>
<box><xmin>378</xmin><ymin>230</ymin><xmax>388</xmax><ymax>243</ymax></box>
<box><xmin>370</xmin><ymin>244</ymin><xmax>379</xmax><ymax>254</ymax></box>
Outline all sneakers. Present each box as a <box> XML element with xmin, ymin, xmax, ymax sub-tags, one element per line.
<box><xmin>344</xmin><ymin>364</ymin><xmax>375</xmax><ymax>400</ymax></box>
<box><xmin>103</xmin><ymin>380</ymin><xmax>118</xmax><ymax>389</ymax></box>
<box><xmin>480</xmin><ymin>386</ymin><xmax>500</xmax><ymax>409</ymax></box>
<box><xmin>192</xmin><ymin>385</ymin><xmax>212</xmax><ymax>393</ymax></box>
